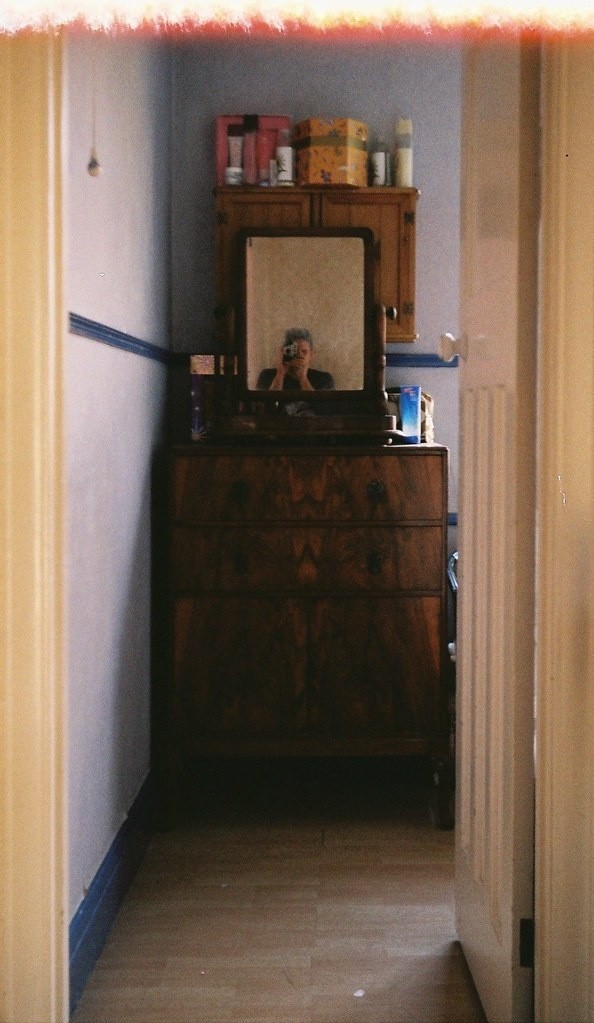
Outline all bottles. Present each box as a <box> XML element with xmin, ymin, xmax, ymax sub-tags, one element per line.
<box><xmin>394</xmin><ymin>120</ymin><xmax>413</xmax><ymax>188</ymax></box>
<box><xmin>371</xmin><ymin>137</ymin><xmax>391</xmax><ymax>186</ymax></box>
<box><xmin>224</xmin><ymin>167</ymin><xmax>242</xmax><ymax>186</ymax></box>
<box><xmin>275</xmin><ymin>129</ymin><xmax>295</xmax><ymax>187</ymax></box>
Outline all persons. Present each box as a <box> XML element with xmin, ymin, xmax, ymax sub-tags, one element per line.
<box><xmin>255</xmin><ymin>328</ymin><xmax>334</xmax><ymax>391</ymax></box>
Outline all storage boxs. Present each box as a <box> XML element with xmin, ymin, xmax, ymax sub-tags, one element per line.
<box><xmin>400</xmin><ymin>385</ymin><xmax>422</xmax><ymax>443</ymax></box>
<box><xmin>213</xmin><ymin>112</ymin><xmax>290</xmax><ymax>183</ymax></box>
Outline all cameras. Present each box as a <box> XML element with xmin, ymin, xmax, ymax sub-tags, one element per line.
<box><xmin>282</xmin><ymin>344</ymin><xmax>297</xmax><ymax>365</ymax></box>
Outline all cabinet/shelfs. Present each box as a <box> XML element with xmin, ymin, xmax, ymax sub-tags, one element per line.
<box><xmin>167</xmin><ymin>442</ymin><xmax>450</xmax><ymax>812</ymax></box>
<box><xmin>213</xmin><ymin>183</ymin><xmax>422</xmax><ymax>346</ymax></box>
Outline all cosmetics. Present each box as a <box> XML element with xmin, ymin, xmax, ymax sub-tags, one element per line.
<box><xmin>398</xmin><ymin>385</ymin><xmax>421</xmax><ymax>444</ymax></box>
<box><xmin>386</xmin><ymin>429</ymin><xmax>418</xmax><ymax>445</ymax></box>
<box><xmin>226</xmin><ymin>112</ymin><xmax>294</xmax><ymax>187</ymax></box>
<box><xmin>369</xmin><ymin>137</ymin><xmax>386</xmax><ymax>186</ymax></box>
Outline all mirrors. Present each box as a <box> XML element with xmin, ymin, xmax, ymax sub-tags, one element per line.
<box><xmin>237</xmin><ymin>226</ymin><xmax>377</xmax><ymax>402</ymax></box>
